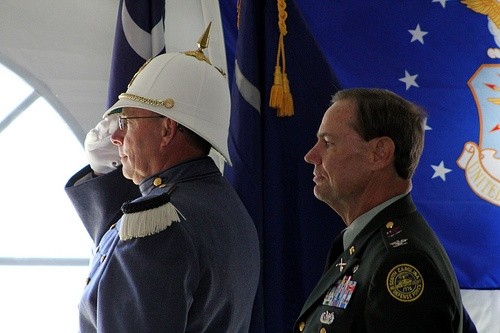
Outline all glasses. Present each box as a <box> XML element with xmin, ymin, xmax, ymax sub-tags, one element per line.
<box><xmin>118</xmin><ymin>115</ymin><xmax>164</xmax><ymax>130</ymax></box>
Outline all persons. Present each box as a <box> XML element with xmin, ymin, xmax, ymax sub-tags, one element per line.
<box><xmin>291</xmin><ymin>89</ymin><xmax>465</xmax><ymax>333</ymax></box>
<box><xmin>65</xmin><ymin>20</ymin><xmax>262</xmax><ymax>333</ymax></box>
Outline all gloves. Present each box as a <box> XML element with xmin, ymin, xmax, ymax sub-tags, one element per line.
<box><xmin>84</xmin><ymin>114</ymin><xmax>122</xmax><ymax>174</ymax></box>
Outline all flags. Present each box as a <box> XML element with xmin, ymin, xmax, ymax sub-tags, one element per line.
<box><xmin>106</xmin><ymin>1</ymin><xmax>478</xmax><ymax>332</ymax></box>
<box><xmin>295</xmin><ymin>0</ymin><xmax>500</xmax><ymax>291</ymax></box>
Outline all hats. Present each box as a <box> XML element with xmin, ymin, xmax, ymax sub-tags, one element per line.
<box><xmin>103</xmin><ymin>21</ymin><xmax>233</xmax><ymax>167</ymax></box>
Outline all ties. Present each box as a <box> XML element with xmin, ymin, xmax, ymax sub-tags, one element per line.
<box><xmin>322</xmin><ymin>229</ymin><xmax>344</xmax><ymax>274</ymax></box>
